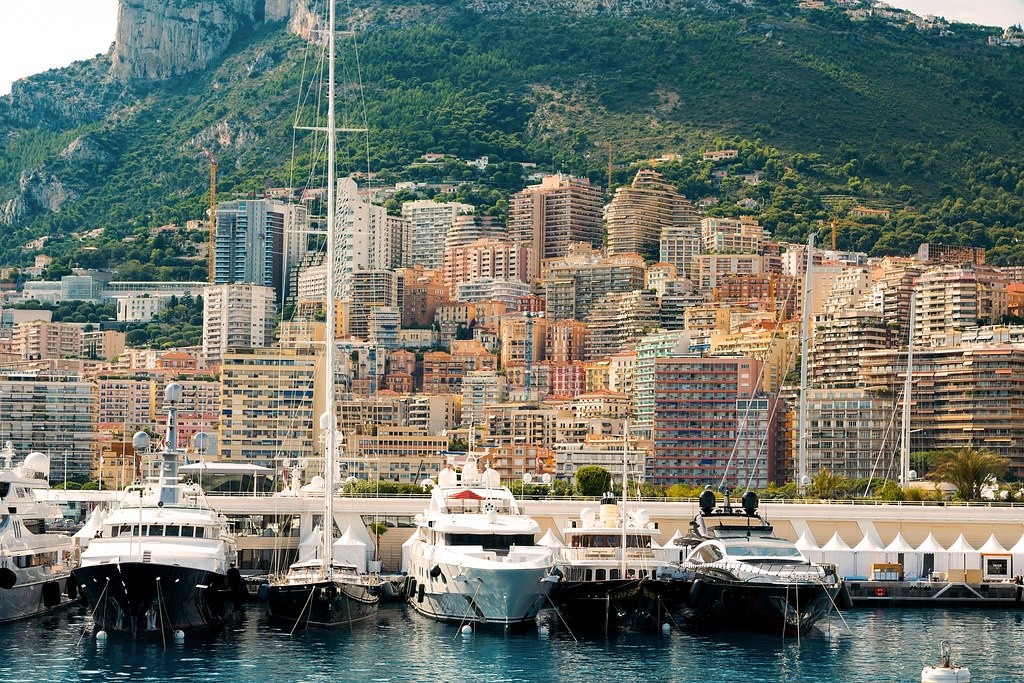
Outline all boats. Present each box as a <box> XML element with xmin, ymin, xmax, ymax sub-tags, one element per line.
<box><xmin>668</xmin><ymin>490</ymin><xmax>844</xmax><ymax>640</ymax></box>
<box><xmin>0</xmin><ymin>440</ymin><xmax>83</xmax><ymax>623</ymax></box>
<box><xmin>557</xmin><ymin>416</ymin><xmax>673</xmax><ymax>634</ymax></box>
<box><xmin>71</xmin><ymin>383</ymin><xmax>248</xmax><ymax>635</ymax></box>
<box><xmin>402</xmin><ymin>419</ymin><xmax>560</xmax><ymax>626</ymax></box>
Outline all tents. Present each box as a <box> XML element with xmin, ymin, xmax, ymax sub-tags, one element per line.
<box><xmin>72</xmin><ymin>505</ymin><xmax>112</xmax><ymax>547</ymax></box>
<box><xmin>647</xmin><ymin>530</ymin><xmax>691</xmax><ymax>562</ymax></box>
<box><xmin>297</xmin><ymin>522</ymin><xmax>367</xmax><ymax>573</ymax></box>
<box><xmin>793</xmin><ymin>531</ymin><xmax>1024</xmax><ymax>580</ymax></box>
<box><xmin>402</xmin><ymin>526</ymin><xmax>430</xmax><ymax>574</ymax></box>
<box><xmin>537</xmin><ymin>526</ymin><xmax>563</xmax><ymax>548</ymax></box>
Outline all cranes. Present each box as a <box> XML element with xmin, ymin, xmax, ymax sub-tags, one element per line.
<box><xmin>483</xmin><ymin>311</ymin><xmax>544</xmax><ymax>400</ymax></box>
<box><xmin>819</xmin><ymin>216</ymin><xmax>868</xmax><ymax>250</ymax></box>
<box><xmin>197</xmin><ymin>140</ymin><xmax>218</xmax><ymax>283</ymax></box>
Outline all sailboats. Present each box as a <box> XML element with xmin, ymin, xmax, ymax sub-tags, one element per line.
<box><xmin>268</xmin><ymin>1</ymin><xmax>385</xmax><ymax>628</ymax></box>
